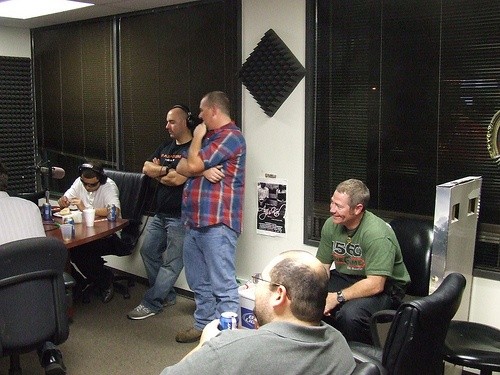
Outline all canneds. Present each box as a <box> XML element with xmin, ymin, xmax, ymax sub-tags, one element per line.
<box><xmin>107</xmin><ymin>204</ymin><xmax>115</xmax><ymax>222</ymax></box>
<box><xmin>64</xmin><ymin>217</ymin><xmax>75</xmax><ymax>237</ymax></box>
<box><xmin>42</xmin><ymin>203</ymin><xmax>52</xmax><ymax>220</ymax></box>
<box><xmin>219</xmin><ymin>311</ymin><xmax>239</xmax><ymax>331</ymax></box>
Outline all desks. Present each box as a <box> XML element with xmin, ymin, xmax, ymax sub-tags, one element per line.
<box><xmin>44</xmin><ymin>214</ymin><xmax>128</xmax><ymax>249</ymax></box>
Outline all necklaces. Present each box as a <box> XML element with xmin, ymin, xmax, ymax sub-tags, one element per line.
<box><xmin>347</xmin><ymin>211</ymin><xmax>365</xmax><ymax>243</ymax></box>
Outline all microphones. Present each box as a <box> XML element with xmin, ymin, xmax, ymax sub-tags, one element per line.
<box><xmin>47</xmin><ymin>166</ymin><xmax>66</xmax><ymax>179</ymax></box>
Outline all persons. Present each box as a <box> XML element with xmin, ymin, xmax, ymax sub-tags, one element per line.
<box><xmin>315</xmin><ymin>178</ymin><xmax>411</xmax><ymax>347</ymax></box>
<box><xmin>126</xmin><ymin>104</ymin><xmax>194</xmax><ymax>319</ymax></box>
<box><xmin>0</xmin><ymin>167</ymin><xmax>67</xmax><ymax>375</ymax></box>
<box><xmin>58</xmin><ymin>160</ymin><xmax>120</xmax><ymax>304</ymax></box>
<box><xmin>259</xmin><ymin>183</ymin><xmax>269</xmax><ymax>208</ymax></box>
<box><xmin>160</xmin><ymin>251</ymin><xmax>356</xmax><ymax>375</ymax></box>
<box><xmin>176</xmin><ymin>91</ymin><xmax>246</xmax><ymax>342</ymax></box>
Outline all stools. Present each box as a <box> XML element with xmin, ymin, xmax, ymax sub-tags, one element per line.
<box><xmin>443</xmin><ymin>321</ymin><xmax>500</xmax><ymax>375</ymax></box>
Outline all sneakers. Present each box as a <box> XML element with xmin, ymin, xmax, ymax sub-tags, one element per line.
<box><xmin>176</xmin><ymin>327</ymin><xmax>202</xmax><ymax>343</ymax></box>
<box><xmin>126</xmin><ymin>304</ymin><xmax>155</xmax><ymax>319</ymax></box>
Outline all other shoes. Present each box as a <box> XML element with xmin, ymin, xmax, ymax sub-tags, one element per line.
<box><xmin>42</xmin><ymin>349</ymin><xmax>66</xmax><ymax>375</ymax></box>
<box><xmin>101</xmin><ymin>281</ymin><xmax>113</xmax><ymax>303</ymax></box>
<box><xmin>72</xmin><ymin>279</ymin><xmax>96</xmax><ymax>300</ymax></box>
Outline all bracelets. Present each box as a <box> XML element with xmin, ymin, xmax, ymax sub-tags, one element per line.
<box><xmin>166</xmin><ymin>167</ymin><xmax>172</xmax><ymax>174</ymax></box>
<box><xmin>160</xmin><ymin>166</ymin><xmax>164</xmax><ymax>176</ymax></box>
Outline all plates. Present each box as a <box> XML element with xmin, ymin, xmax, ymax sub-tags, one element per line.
<box><xmin>53</xmin><ymin>211</ymin><xmax>82</xmax><ymax>217</ymax></box>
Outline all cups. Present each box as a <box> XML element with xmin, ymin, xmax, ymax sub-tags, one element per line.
<box><xmin>83</xmin><ymin>208</ymin><xmax>96</xmax><ymax>227</ymax></box>
<box><xmin>71</xmin><ymin>213</ymin><xmax>83</xmax><ymax>223</ymax></box>
<box><xmin>238</xmin><ymin>283</ymin><xmax>255</xmax><ymax>329</ymax></box>
<box><xmin>60</xmin><ymin>224</ymin><xmax>73</xmax><ymax>241</ymax></box>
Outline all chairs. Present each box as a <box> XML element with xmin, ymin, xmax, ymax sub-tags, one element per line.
<box><xmin>349</xmin><ymin>217</ymin><xmax>466</xmax><ymax>375</ymax></box>
<box><xmin>1</xmin><ymin>237</ymin><xmax>76</xmax><ymax>374</ymax></box>
<box><xmin>79</xmin><ymin>169</ymin><xmax>149</xmax><ymax>304</ymax></box>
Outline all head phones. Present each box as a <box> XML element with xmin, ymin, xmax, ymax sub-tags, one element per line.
<box><xmin>78</xmin><ymin>163</ymin><xmax>107</xmax><ymax>184</ymax></box>
<box><xmin>173</xmin><ymin>105</ymin><xmax>196</xmax><ymax>128</ymax></box>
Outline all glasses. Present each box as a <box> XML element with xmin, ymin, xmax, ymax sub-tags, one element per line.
<box><xmin>251</xmin><ymin>272</ymin><xmax>291</xmax><ymax>300</ymax></box>
<box><xmin>80</xmin><ymin>174</ymin><xmax>100</xmax><ymax>188</ymax></box>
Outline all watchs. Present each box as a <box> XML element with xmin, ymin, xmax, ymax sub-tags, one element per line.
<box><xmin>337</xmin><ymin>291</ymin><xmax>346</xmax><ymax>305</ymax></box>
